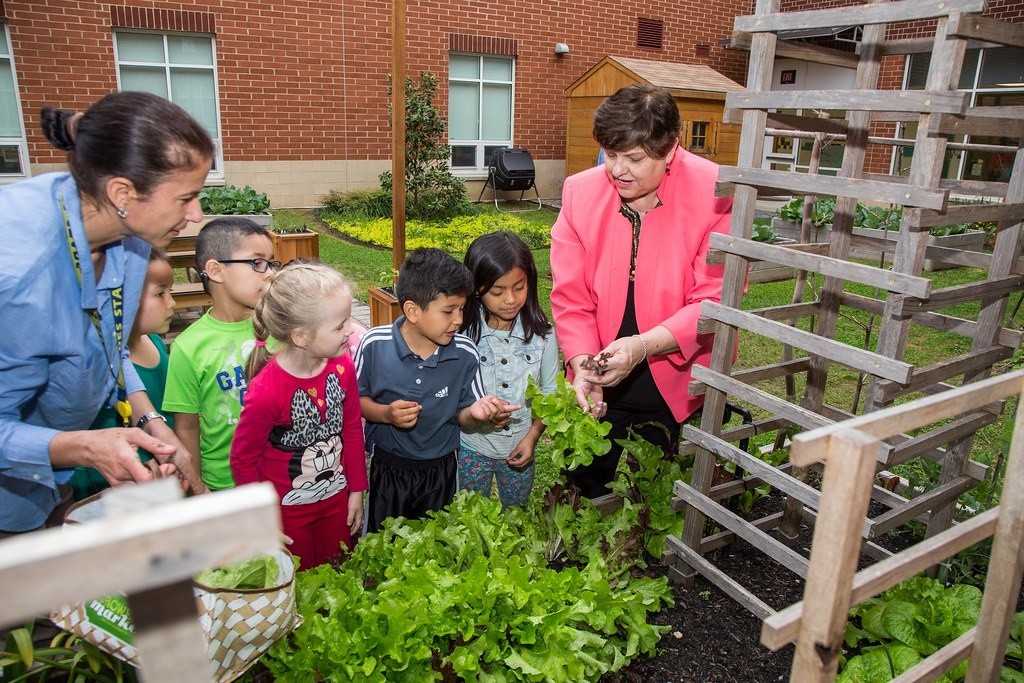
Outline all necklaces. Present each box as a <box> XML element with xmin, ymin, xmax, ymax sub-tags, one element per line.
<box><xmin>57</xmin><ymin>189</ymin><xmax>132</xmax><ymax>427</ymax></box>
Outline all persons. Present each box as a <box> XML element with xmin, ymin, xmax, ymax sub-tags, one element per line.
<box><xmin>268</xmin><ymin>253</ymin><xmax>369</xmax><ymax>534</ymax></box>
<box><xmin>450</xmin><ymin>231</ymin><xmax>559</xmax><ymax>513</ymax></box>
<box><xmin>161</xmin><ymin>216</ymin><xmax>292</xmax><ymax>493</ymax></box>
<box><xmin>0</xmin><ymin>89</ymin><xmax>214</xmax><ymax>537</ymax></box>
<box><xmin>351</xmin><ymin>246</ymin><xmax>522</xmax><ymax>538</ymax></box>
<box><xmin>229</xmin><ymin>261</ymin><xmax>371</xmax><ymax>571</ymax></box>
<box><xmin>67</xmin><ymin>246</ymin><xmax>179</xmax><ymax>520</ymax></box>
<box><xmin>548</xmin><ymin>83</ymin><xmax>752</xmax><ymax>497</ymax></box>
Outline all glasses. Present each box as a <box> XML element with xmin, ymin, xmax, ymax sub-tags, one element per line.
<box><xmin>217</xmin><ymin>258</ymin><xmax>282</xmax><ymax>273</ymax></box>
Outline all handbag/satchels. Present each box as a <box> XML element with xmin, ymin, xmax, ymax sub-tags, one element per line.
<box><xmin>50</xmin><ymin>458</ymin><xmax>305</xmax><ymax>683</ymax></box>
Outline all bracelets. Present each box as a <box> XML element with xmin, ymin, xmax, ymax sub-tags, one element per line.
<box><xmin>136</xmin><ymin>409</ymin><xmax>168</xmax><ymax>429</ymax></box>
<box><xmin>633</xmin><ymin>333</ymin><xmax>650</xmax><ymax>364</ymax></box>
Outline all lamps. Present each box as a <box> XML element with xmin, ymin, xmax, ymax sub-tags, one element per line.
<box><xmin>556</xmin><ymin>42</ymin><xmax>571</xmax><ymax>54</ymax></box>
